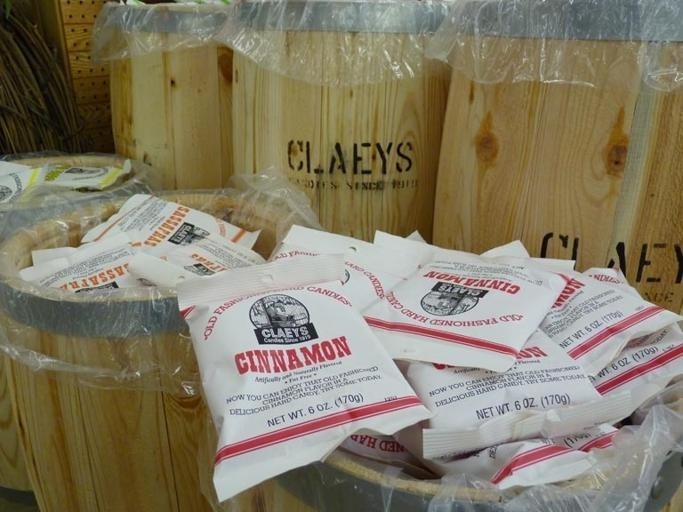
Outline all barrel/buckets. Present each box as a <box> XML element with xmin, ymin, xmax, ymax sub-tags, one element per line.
<box><xmin>265</xmin><ymin>396</ymin><xmax>661</xmax><ymax>512</ymax></box>
<box><xmin>432</xmin><ymin>0</ymin><xmax>683</xmax><ymax>316</ymax></box>
<box><xmin>230</xmin><ymin>0</ymin><xmax>453</xmax><ymax>244</ymax></box>
<box><xmin>0</xmin><ymin>191</ymin><xmax>292</xmax><ymax>512</ymax></box>
<box><xmin>104</xmin><ymin>0</ymin><xmax>235</xmax><ymax>196</ymax></box>
<box><xmin>0</xmin><ymin>154</ymin><xmax>149</xmax><ymax>504</ymax></box>
